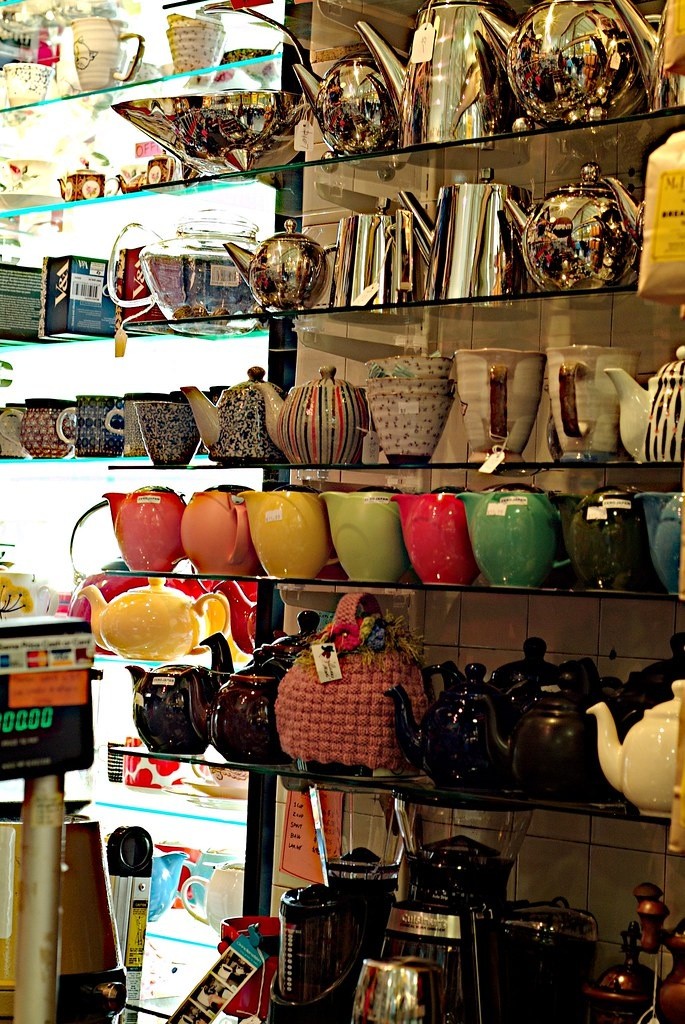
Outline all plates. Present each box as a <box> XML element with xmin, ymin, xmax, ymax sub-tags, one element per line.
<box><xmin>1</xmin><ymin>194</ymin><xmax>55</xmax><ymax>211</ymax></box>
<box><xmin>184</xmin><ymin>782</ymin><xmax>250</xmax><ymax>802</ymax></box>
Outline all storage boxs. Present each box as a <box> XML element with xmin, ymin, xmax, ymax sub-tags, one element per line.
<box><xmin>44</xmin><ymin>256</ymin><xmax>115</xmax><ymax>338</ymax></box>
<box><xmin>180</xmin><ymin>256</ymin><xmax>255</xmax><ymax>315</ymax></box>
<box><xmin>0</xmin><ymin>262</ymin><xmax>42</xmax><ymax>338</ymax></box>
<box><xmin>114</xmin><ymin>248</ymin><xmax>186</xmax><ymax>326</ymax></box>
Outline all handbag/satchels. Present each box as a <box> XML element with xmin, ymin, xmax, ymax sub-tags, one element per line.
<box><xmin>275</xmin><ymin>589</ymin><xmax>425</xmax><ymax>773</ymax></box>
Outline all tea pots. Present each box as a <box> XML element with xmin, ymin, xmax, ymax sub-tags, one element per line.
<box><xmin>180</xmin><ymin>364</ymin><xmax>368</xmax><ymax>468</ymax></box>
<box><xmin>150</xmin><ymin>837</ymin><xmax>246</xmax><ymax>936</ymax></box>
<box><xmin>57</xmin><ymin>149</ymin><xmax>177</xmax><ymax>202</ymax></box>
<box><xmin>604</xmin><ymin>345</ymin><xmax>685</xmax><ymax>463</ymax></box>
<box><xmin>292</xmin><ymin>0</ymin><xmax>685</xmax><ymax>168</ymax></box>
<box><xmin>383</xmin><ymin>630</ymin><xmax>685</xmax><ymax>817</ymax></box>
<box><xmin>77</xmin><ymin>577</ymin><xmax>320</xmax><ymax>765</ymax></box>
<box><xmin>107</xmin><ymin>163</ymin><xmax>642</xmax><ymax>335</ymax></box>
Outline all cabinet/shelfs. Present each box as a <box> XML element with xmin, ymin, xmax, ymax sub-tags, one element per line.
<box><xmin>0</xmin><ymin>0</ymin><xmax>684</xmax><ymax>1024</ymax></box>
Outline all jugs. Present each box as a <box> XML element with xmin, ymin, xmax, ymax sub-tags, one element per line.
<box><xmin>102</xmin><ymin>478</ymin><xmax>684</xmax><ymax>598</ymax></box>
<box><xmin>348</xmin><ymin>954</ymin><xmax>449</xmax><ymax>1024</ymax></box>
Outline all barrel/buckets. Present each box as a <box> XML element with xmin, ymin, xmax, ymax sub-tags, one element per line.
<box><xmin>220</xmin><ymin>913</ymin><xmax>281</xmax><ymax>1020</ymax></box>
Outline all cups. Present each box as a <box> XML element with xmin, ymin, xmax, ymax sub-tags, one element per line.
<box><xmin>2</xmin><ymin>14</ymin><xmax>226</xmax><ymax>107</ymax></box>
<box><xmin>7</xmin><ymin>159</ymin><xmax>51</xmax><ymax>192</ymax></box>
<box><xmin>365</xmin><ymin>345</ymin><xmax>641</xmax><ymax>463</ymax></box>
<box><xmin>105</xmin><ymin>734</ymin><xmax>250</xmax><ymax>786</ymax></box>
<box><xmin>0</xmin><ymin>391</ymin><xmax>202</xmax><ymax>467</ymax></box>
